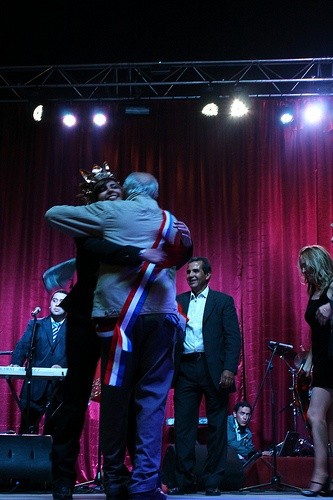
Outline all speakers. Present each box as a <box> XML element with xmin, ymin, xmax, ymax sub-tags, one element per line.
<box><xmin>0</xmin><ymin>433</ymin><xmax>54</xmax><ymax>494</ymax></box>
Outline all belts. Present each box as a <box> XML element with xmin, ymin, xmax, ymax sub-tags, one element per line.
<box><xmin>180</xmin><ymin>353</ymin><xmax>206</xmax><ymax>363</ymax></box>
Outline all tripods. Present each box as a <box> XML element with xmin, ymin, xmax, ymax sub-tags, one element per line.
<box><xmin>240</xmin><ymin>342</ymin><xmax>304</xmax><ymax>492</ymax></box>
<box><xmin>74</xmin><ymin>447</ymin><xmax>106</xmax><ymax>495</ymax></box>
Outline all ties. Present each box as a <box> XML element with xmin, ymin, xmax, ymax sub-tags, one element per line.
<box><xmin>52</xmin><ymin>321</ymin><xmax>62</xmax><ymax>342</ymax></box>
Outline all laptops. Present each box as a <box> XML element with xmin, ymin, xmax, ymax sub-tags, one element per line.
<box><xmin>276</xmin><ymin>431</ymin><xmax>299</xmax><ymax>457</ymax></box>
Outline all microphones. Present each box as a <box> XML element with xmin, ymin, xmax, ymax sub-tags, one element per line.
<box><xmin>269</xmin><ymin>341</ymin><xmax>294</xmax><ymax>350</ymax></box>
<box><xmin>31</xmin><ymin>307</ymin><xmax>41</xmax><ymax>317</ymax></box>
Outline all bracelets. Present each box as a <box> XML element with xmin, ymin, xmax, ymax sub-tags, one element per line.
<box><xmin>140</xmin><ymin>247</ymin><xmax>146</xmax><ymax>256</ymax></box>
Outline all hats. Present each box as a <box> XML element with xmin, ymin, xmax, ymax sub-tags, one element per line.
<box><xmin>80</xmin><ymin>160</ymin><xmax>117</xmax><ymax>204</ymax></box>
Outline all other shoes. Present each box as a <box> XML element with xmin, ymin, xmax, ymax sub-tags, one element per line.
<box><xmin>206</xmin><ymin>485</ymin><xmax>221</xmax><ymax>496</ymax></box>
<box><xmin>53</xmin><ymin>486</ymin><xmax>71</xmax><ymax>500</ymax></box>
<box><xmin>129</xmin><ymin>489</ymin><xmax>166</xmax><ymax>500</ymax></box>
<box><xmin>168</xmin><ymin>486</ymin><xmax>197</xmax><ymax>495</ymax></box>
<box><xmin>106</xmin><ymin>484</ymin><xmax>129</xmax><ymax>500</ymax></box>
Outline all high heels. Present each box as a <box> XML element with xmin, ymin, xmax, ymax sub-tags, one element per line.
<box><xmin>318</xmin><ymin>480</ymin><xmax>333</xmax><ymax>497</ymax></box>
<box><xmin>301</xmin><ymin>474</ymin><xmax>330</xmax><ymax>497</ymax></box>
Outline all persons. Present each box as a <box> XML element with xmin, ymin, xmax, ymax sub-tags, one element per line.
<box><xmin>298</xmin><ymin>244</ymin><xmax>333</xmax><ymax>497</ymax></box>
<box><xmin>9</xmin><ymin>289</ymin><xmax>69</xmax><ymax>434</ymax></box>
<box><xmin>166</xmin><ymin>257</ymin><xmax>241</xmax><ymax>496</ymax></box>
<box><xmin>45</xmin><ymin>172</ymin><xmax>180</xmax><ymax>500</ymax></box>
<box><xmin>51</xmin><ymin>161</ymin><xmax>194</xmax><ymax>500</ymax></box>
<box><xmin>227</xmin><ymin>401</ymin><xmax>255</xmax><ymax>460</ymax></box>
